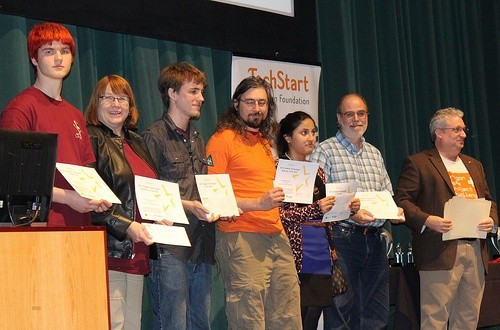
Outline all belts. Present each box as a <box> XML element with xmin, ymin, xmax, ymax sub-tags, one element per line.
<box><xmin>460</xmin><ymin>238</ymin><xmax>477</xmax><ymax>242</ymax></box>
<box><xmin>338</xmin><ymin>221</ymin><xmax>381</xmax><ymax>235</ymax></box>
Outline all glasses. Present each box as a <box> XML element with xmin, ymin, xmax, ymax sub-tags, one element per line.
<box><xmin>339</xmin><ymin>111</ymin><xmax>369</xmax><ymax>118</ymax></box>
<box><xmin>437</xmin><ymin>126</ymin><xmax>470</xmax><ymax>132</ymax></box>
<box><xmin>238</xmin><ymin>98</ymin><xmax>270</xmax><ymax>107</ymax></box>
<box><xmin>98</xmin><ymin>95</ymin><xmax>130</xmax><ymax>103</ymax></box>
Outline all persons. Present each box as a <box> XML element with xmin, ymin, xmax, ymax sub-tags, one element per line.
<box><xmin>0</xmin><ymin>21</ymin><xmax>113</xmax><ymax>228</ymax></box>
<box><xmin>205</xmin><ymin>76</ymin><xmax>305</xmax><ymax>330</ymax></box>
<box><xmin>394</xmin><ymin>106</ymin><xmax>499</xmax><ymax>330</ymax></box>
<box><xmin>136</xmin><ymin>61</ymin><xmax>244</xmax><ymax>330</ymax></box>
<box><xmin>276</xmin><ymin>111</ymin><xmax>360</xmax><ymax>330</ymax></box>
<box><xmin>83</xmin><ymin>73</ymin><xmax>174</xmax><ymax>330</ymax></box>
<box><xmin>307</xmin><ymin>91</ymin><xmax>406</xmax><ymax>330</ymax></box>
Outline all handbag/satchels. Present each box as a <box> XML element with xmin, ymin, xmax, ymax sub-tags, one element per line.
<box><xmin>328</xmin><ymin>248</ymin><xmax>347</xmax><ymax>297</ymax></box>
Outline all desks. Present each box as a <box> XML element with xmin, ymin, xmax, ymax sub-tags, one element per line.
<box><xmin>0</xmin><ymin>221</ymin><xmax>111</xmax><ymax>330</ymax></box>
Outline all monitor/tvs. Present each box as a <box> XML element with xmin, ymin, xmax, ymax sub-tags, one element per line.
<box><xmin>0</xmin><ymin>129</ymin><xmax>58</xmax><ymax>227</ymax></box>
